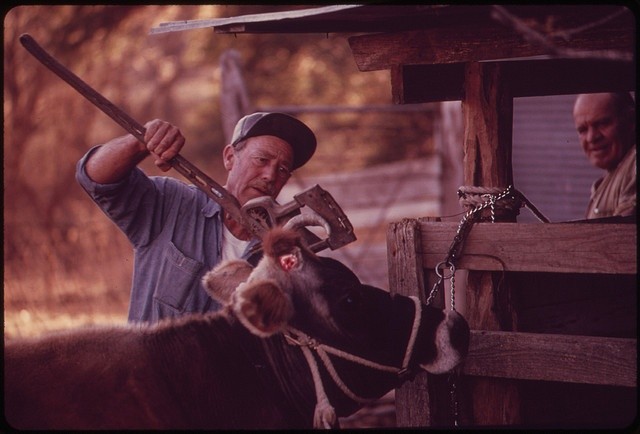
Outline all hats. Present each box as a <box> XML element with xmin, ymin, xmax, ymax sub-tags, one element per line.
<box><xmin>230</xmin><ymin>112</ymin><xmax>316</xmax><ymax>169</ymax></box>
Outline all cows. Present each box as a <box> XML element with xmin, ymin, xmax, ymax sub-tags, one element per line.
<box><xmin>2</xmin><ymin>225</ymin><xmax>473</xmax><ymax>430</ymax></box>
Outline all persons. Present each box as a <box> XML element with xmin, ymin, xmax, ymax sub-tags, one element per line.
<box><xmin>74</xmin><ymin>111</ymin><xmax>317</xmax><ymax>325</ymax></box>
<box><xmin>572</xmin><ymin>93</ymin><xmax>640</xmax><ymax>220</ymax></box>
